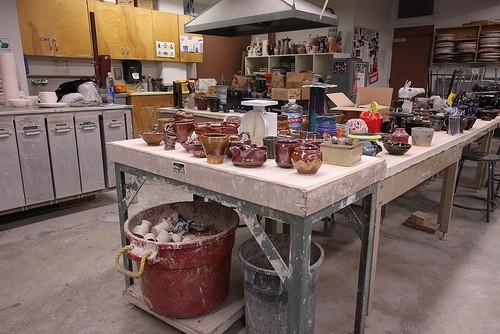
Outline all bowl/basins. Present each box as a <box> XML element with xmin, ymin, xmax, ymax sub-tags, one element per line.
<box><xmin>138</xmin><ymin>133</ymin><xmax>165</xmax><ymax>147</ymax></box>
<box><xmin>347</xmin><ymin>134</ymin><xmax>382</xmax><ymax>155</ymax></box>
<box><xmin>411</xmin><ymin>127</ymin><xmax>435</xmax><ymax>146</ymax></box>
<box><xmin>429</xmin><ymin>109</ymin><xmax>500</xmax><ymax>131</ymax></box>
<box><xmin>180</xmin><ymin>142</ymin><xmax>204</xmax><ymax>152</ymax></box>
<box><xmin>383</xmin><ymin>143</ymin><xmax>412</xmax><ymax>155</ymax></box>
<box><xmin>7</xmin><ymin>99</ymin><xmax>29</xmax><ymax>107</ymax></box>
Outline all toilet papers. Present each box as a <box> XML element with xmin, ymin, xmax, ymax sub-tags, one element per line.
<box><xmin>0</xmin><ymin>52</ymin><xmax>19</xmax><ymax>107</ymax></box>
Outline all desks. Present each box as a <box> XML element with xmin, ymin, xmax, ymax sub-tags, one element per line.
<box><xmin>106</xmin><ymin>107</ymin><xmax>500</xmax><ymax>334</ymax></box>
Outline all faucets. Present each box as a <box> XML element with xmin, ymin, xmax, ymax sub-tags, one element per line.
<box><xmin>132</xmin><ymin>81</ymin><xmax>142</xmax><ymax>92</ymax></box>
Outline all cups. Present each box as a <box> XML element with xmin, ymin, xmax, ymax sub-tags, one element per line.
<box><xmin>197</xmin><ymin>96</ymin><xmax>230</xmax><ymax>113</ymax></box>
<box><xmin>153</xmin><ymin>82</ymin><xmax>161</xmax><ymax>91</ymax></box>
<box><xmin>142</xmin><ymin>83</ymin><xmax>148</xmax><ymax>91</ymax></box>
<box><xmin>159</xmin><ymin>118</ymin><xmax>229</xmax><ymax>164</ymax></box>
<box><xmin>36</xmin><ymin>92</ymin><xmax>57</xmax><ymax>103</ymax></box>
<box><xmin>263</xmin><ymin>131</ymin><xmax>325</xmax><ymax>173</ymax></box>
<box><xmin>20</xmin><ymin>96</ymin><xmax>38</xmax><ymax>106</ymax></box>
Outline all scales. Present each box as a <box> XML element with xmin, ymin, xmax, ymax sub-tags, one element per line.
<box><xmin>238</xmin><ymin>99</ymin><xmax>278</xmax><ymax>147</ymax></box>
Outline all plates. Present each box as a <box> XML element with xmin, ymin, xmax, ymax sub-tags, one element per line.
<box><xmin>38</xmin><ymin>103</ymin><xmax>67</xmax><ymax>108</ymax></box>
<box><xmin>433</xmin><ymin>31</ymin><xmax>500</xmax><ymax>63</ymax></box>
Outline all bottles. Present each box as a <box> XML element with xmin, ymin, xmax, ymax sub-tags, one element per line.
<box><xmin>106</xmin><ymin>72</ymin><xmax>116</xmax><ymax>105</ymax></box>
<box><xmin>449</xmin><ymin>103</ymin><xmax>460</xmax><ymax>136</ymax></box>
<box><xmin>281</xmin><ymin>98</ymin><xmax>303</xmax><ymax>135</ymax></box>
<box><xmin>391</xmin><ymin>128</ymin><xmax>409</xmax><ymax>144</ymax></box>
<box><xmin>254</xmin><ymin>37</ymin><xmax>289</xmax><ymax>56</ymax></box>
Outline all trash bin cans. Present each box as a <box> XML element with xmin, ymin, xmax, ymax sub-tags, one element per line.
<box><xmin>124</xmin><ymin>200</ymin><xmax>240</xmax><ymax>318</ymax></box>
<box><xmin>238</xmin><ymin>233</ymin><xmax>324</xmax><ymax>334</ymax></box>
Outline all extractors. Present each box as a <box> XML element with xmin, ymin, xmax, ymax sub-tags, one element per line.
<box><xmin>184</xmin><ymin>0</ymin><xmax>345</xmax><ymax>38</ymax></box>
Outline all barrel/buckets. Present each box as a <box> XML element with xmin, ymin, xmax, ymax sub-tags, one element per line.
<box><xmin>115</xmin><ymin>201</ymin><xmax>240</xmax><ymax>319</ymax></box>
<box><xmin>239</xmin><ymin>234</ymin><xmax>325</xmax><ymax>333</ymax></box>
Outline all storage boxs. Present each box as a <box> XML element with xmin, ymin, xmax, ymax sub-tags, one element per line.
<box><xmin>325</xmin><ymin>87</ymin><xmax>394</xmax><ymax>123</ymax></box>
<box><xmin>272</xmin><ymin>71</ymin><xmax>316</xmax><ymax>100</ymax></box>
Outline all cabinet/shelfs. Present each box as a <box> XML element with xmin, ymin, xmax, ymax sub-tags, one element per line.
<box><xmin>245</xmin><ymin>52</ymin><xmax>351</xmax><ymax>108</ymax></box>
<box><xmin>125</xmin><ymin>93</ymin><xmax>198</xmax><ymax>139</ymax></box>
<box><xmin>431</xmin><ymin>23</ymin><xmax>500</xmax><ymax>65</ymax></box>
<box><xmin>0</xmin><ymin>104</ymin><xmax>134</xmax><ymax>215</ymax></box>
<box><xmin>16</xmin><ymin>0</ymin><xmax>203</xmax><ymax>64</ymax></box>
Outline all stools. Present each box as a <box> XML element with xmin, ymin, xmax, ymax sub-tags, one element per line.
<box><xmin>452</xmin><ymin>151</ymin><xmax>500</xmax><ymax>223</ymax></box>
